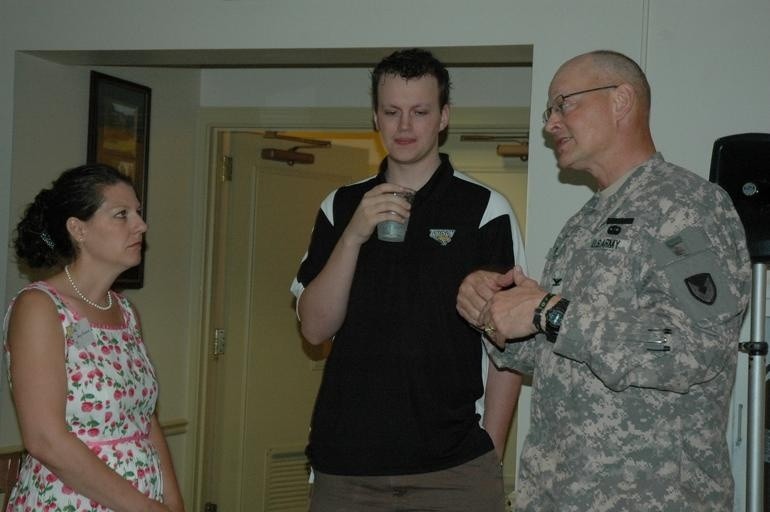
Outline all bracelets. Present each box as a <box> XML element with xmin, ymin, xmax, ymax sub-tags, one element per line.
<box><xmin>532</xmin><ymin>288</ymin><xmax>555</xmax><ymax>338</ymax></box>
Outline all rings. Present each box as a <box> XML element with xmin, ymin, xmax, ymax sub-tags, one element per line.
<box><xmin>483</xmin><ymin>322</ymin><xmax>497</xmax><ymax>335</ymax></box>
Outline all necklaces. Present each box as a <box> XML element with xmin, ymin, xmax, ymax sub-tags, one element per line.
<box><xmin>63</xmin><ymin>263</ymin><xmax>113</xmax><ymax>312</ymax></box>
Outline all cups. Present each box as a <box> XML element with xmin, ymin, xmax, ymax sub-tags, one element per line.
<box><xmin>375</xmin><ymin>186</ymin><xmax>419</xmax><ymax>243</ymax></box>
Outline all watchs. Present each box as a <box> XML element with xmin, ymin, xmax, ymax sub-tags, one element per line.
<box><xmin>543</xmin><ymin>298</ymin><xmax>571</xmax><ymax>342</ymax></box>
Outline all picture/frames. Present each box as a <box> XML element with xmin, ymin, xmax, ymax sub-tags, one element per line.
<box><xmin>82</xmin><ymin>69</ymin><xmax>152</xmax><ymax>292</ymax></box>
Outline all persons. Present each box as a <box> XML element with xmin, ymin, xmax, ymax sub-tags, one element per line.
<box><xmin>7</xmin><ymin>165</ymin><xmax>186</xmax><ymax>512</ymax></box>
<box><xmin>296</xmin><ymin>48</ymin><xmax>525</xmax><ymax>511</ymax></box>
<box><xmin>456</xmin><ymin>50</ymin><xmax>750</xmax><ymax>512</ymax></box>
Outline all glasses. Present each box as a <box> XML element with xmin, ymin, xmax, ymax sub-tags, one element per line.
<box><xmin>541</xmin><ymin>84</ymin><xmax>617</xmax><ymax>124</ymax></box>
